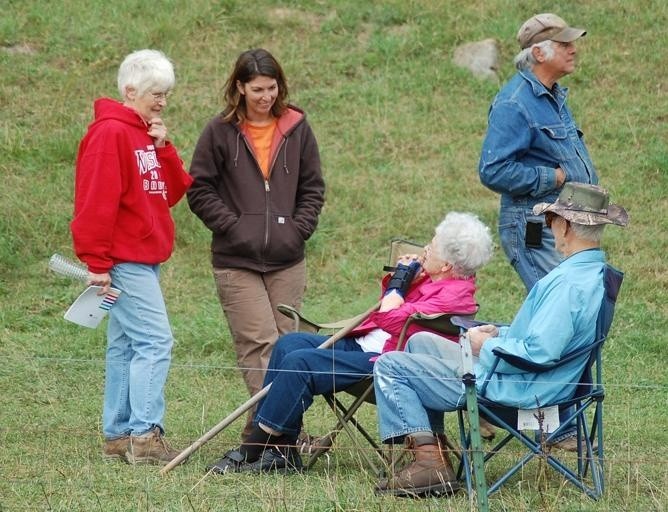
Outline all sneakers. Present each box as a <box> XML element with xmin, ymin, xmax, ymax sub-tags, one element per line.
<box><xmin>102</xmin><ymin>424</ymin><xmax>190</xmax><ymax>466</ymax></box>
<box><xmin>240</xmin><ymin>411</ymin><xmax>335</xmax><ymax>452</ymax></box>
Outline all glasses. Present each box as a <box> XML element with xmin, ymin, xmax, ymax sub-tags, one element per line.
<box><xmin>145</xmin><ymin>88</ymin><xmax>170</xmax><ymax>97</ymax></box>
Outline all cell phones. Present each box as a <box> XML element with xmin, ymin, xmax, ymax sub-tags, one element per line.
<box><xmin>525</xmin><ymin>220</ymin><xmax>543</xmax><ymax>249</ymax></box>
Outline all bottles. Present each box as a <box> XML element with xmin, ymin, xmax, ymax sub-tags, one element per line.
<box><xmin>47</xmin><ymin>254</ymin><xmax>90</xmax><ymax>280</ymax></box>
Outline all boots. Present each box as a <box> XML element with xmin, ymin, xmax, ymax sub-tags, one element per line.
<box><xmin>374</xmin><ymin>437</ymin><xmax>461</xmax><ymax>499</ymax></box>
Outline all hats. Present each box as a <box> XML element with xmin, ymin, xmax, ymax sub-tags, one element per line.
<box><xmin>531</xmin><ymin>181</ymin><xmax>630</xmax><ymax>228</ymax></box>
<box><xmin>515</xmin><ymin>12</ymin><xmax>588</xmax><ymax>51</ymax></box>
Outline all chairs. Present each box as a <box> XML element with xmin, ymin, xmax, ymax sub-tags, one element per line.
<box><xmin>450</xmin><ymin>264</ymin><xmax>624</xmax><ymax>512</ymax></box>
<box><xmin>277</xmin><ymin>239</ymin><xmax>481</xmax><ymax>477</ymax></box>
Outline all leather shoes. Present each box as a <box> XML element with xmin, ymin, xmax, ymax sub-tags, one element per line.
<box><xmin>206</xmin><ymin>443</ymin><xmax>303</xmax><ymax>477</ymax></box>
<box><xmin>552</xmin><ymin>432</ymin><xmax>600</xmax><ymax>456</ymax></box>
<box><xmin>463</xmin><ymin>409</ymin><xmax>496</xmax><ymax>441</ymax></box>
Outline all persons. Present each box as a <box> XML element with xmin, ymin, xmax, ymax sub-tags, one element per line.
<box><xmin>372</xmin><ymin>180</ymin><xmax>630</xmax><ymax>497</ymax></box>
<box><xmin>186</xmin><ymin>47</ymin><xmax>326</xmax><ymax>455</ymax></box>
<box><xmin>69</xmin><ymin>48</ymin><xmax>194</xmax><ymax>467</ymax></box>
<box><xmin>203</xmin><ymin>210</ymin><xmax>494</xmax><ymax>477</ymax></box>
<box><xmin>478</xmin><ymin>13</ymin><xmax>600</xmax><ymax>455</ymax></box>
